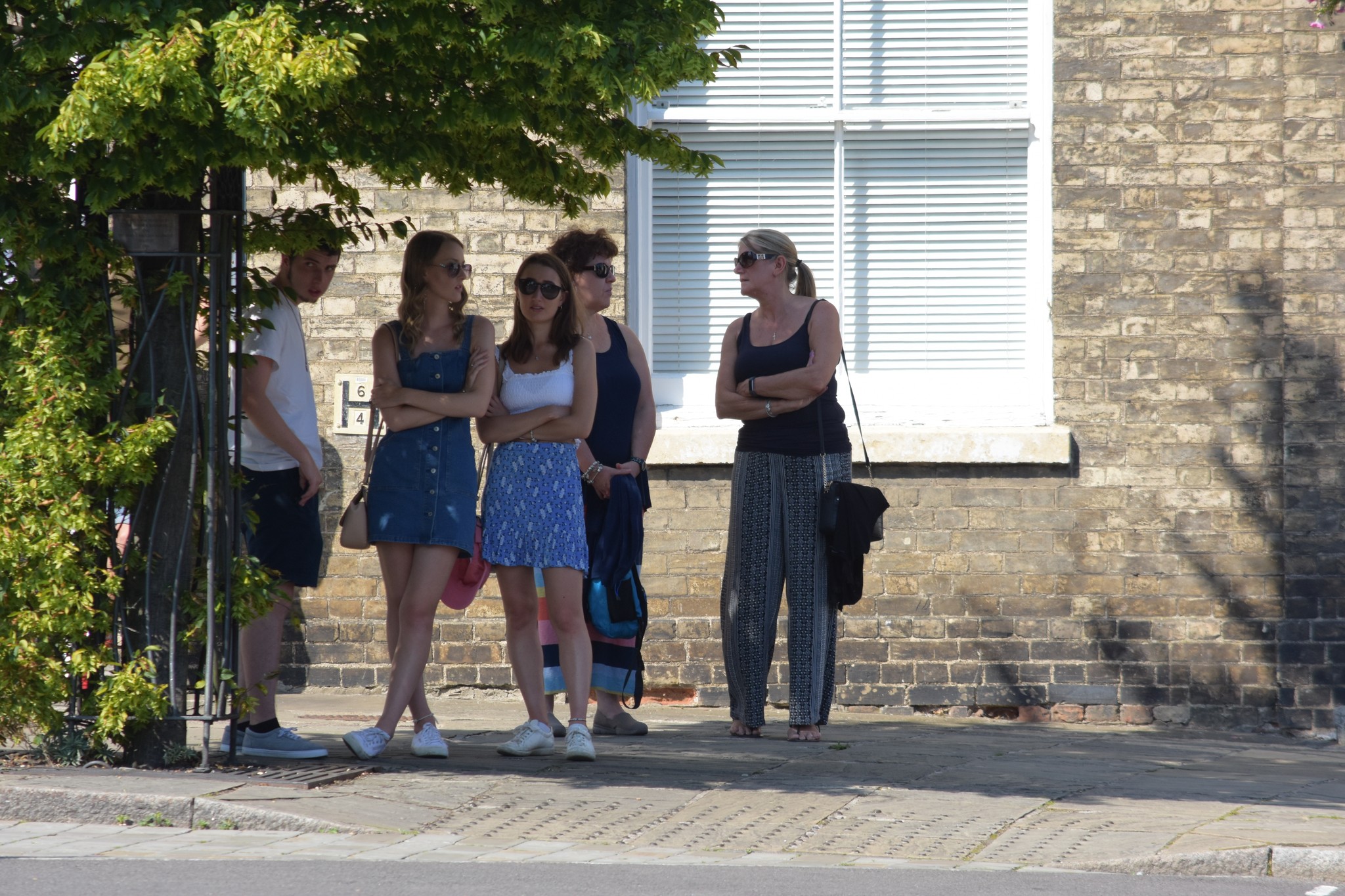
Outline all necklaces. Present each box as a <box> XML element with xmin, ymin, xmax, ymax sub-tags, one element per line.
<box><xmin>760</xmin><ymin>308</ymin><xmax>787</xmax><ymax>340</ymax></box>
<box><xmin>581</xmin><ymin>320</ymin><xmax>599</xmax><ymax>340</ymax></box>
<box><xmin>534</xmin><ymin>343</ymin><xmax>555</xmax><ymax>360</ymax></box>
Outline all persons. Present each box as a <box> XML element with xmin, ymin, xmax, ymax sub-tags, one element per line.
<box><xmin>715</xmin><ymin>230</ymin><xmax>854</xmax><ymax>742</ymax></box>
<box><xmin>342</xmin><ymin>231</ymin><xmax>499</xmax><ymax>760</ymax></box>
<box><xmin>534</xmin><ymin>231</ymin><xmax>659</xmax><ymax>736</ymax></box>
<box><xmin>220</xmin><ymin>217</ymin><xmax>341</xmax><ymax>759</ymax></box>
<box><xmin>476</xmin><ymin>253</ymin><xmax>600</xmax><ymax>760</ymax></box>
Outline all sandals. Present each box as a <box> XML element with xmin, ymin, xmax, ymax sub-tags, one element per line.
<box><xmin>730</xmin><ymin>716</ymin><xmax>764</xmax><ymax>737</ymax></box>
<box><xmin>787</xmin><ymin>723</ymin><xmax>821</xmax><ymax>742</ymax></box>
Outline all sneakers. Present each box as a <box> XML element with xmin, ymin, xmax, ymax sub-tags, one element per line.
<box><xmin>564</xmin><ymin>727</ymin><xmax>597</xmax><ymax>760</ymax></box>
<box><xmin>496</xmin><ymin>720</ymin><xmax>555</xmax><ymax>756</ymax></box>
<box><xmin>220</xmin><ymin>726</ymin><xmax>247</xmax><ymax>755</ymax></box>
<box><xmin>241</xmin><ymin>725</ymin><xmax>328</xmax><ymax>759</ymax></box>
<box><xmin>410</xmin><ymin>722</ymin><xmax>449</xmax><ymax>758</ymax></box>
<box><xmin>343</xmin><ymin>726</ymin><xmax>390</xmax><ymax>760</ymax></box>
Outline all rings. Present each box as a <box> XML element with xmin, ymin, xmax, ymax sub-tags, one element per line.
<box><xmin>471</xmin><ymin>364</ymin><xmax>475</xmax><ymax>367</ymax></box>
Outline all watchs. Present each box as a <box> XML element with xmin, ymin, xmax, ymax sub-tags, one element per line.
<box><xmin>630</xmin><ymin>457</ymin><xmax>648</xmax><ymax>472</ymax></box>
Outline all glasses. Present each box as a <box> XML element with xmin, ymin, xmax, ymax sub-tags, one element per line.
<box><xmin>518</xmin><ymin>277</ymin><xmax>565</xmax><ymax>301</ymax></box>
<box><xmin>577</xmin><ymin>263</ymin><xmax>615</xmax><ymax>279</ymax></box>
<box><xmin>733</xmin><ymin>251</ymin><xmax>777</xmax><ymax>268</ymax></box>
<box><xmin>425</xmin><ymin>261</ymin><xmax>472</xmax><ymax>281</ymax></box>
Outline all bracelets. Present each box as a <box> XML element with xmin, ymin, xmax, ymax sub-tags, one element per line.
<box><xmin>581</xmin><ymin>459</ymin><xmax>604</xmax><ymax>486</ymax></box>
<box><xmin>519</xmin><ymin>436</ymin><xmax>525</xmax><ymax>440</ymax></box>
<box><xmin>460</xmin><ymin>387</ymin><xmax>466</xmax><ymax>392</ymax></box>
<box><xmin>764</xmin><ymin>399</ymin><xmax>777</xmax><ymax>418</ymax></box>
<box><xmin>747</xmin><ymin>377</ymin><xmax>762</xmax><ymax>398</ymax></box>
<box><xmin>530</xmin><ymin>430</ymin><xmax>537</xmax><ymax>442</ymax></box>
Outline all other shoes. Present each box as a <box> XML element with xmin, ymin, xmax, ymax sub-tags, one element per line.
<box><xmin>591</xmin><ymin>709</ymin><xmax>648</xmax><ymax>737</ymax></box>
<box><xmin>527</xmin><ymin>714</ymin><xmax>566</xmax><ymax>737</ymax></box>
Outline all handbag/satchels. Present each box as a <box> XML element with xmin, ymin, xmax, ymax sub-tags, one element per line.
<box><xmin>438</xmin><ymin>514</ymin><xmax>490</xmax><ymax>611</ymax></box>
<box><xmin>339</xmin><ymin>480</ymin><xmax>371</xmax><ymax>550</ymax></box>
<box><xmin>589</xmin><ymin>562</ymin><xmax>648</xmax><ymax>639</ymax></box>
<box><xmin>819</xmin><ymin>479</ymin><xmax>884</xmax><ymax>542</ymax></box>
